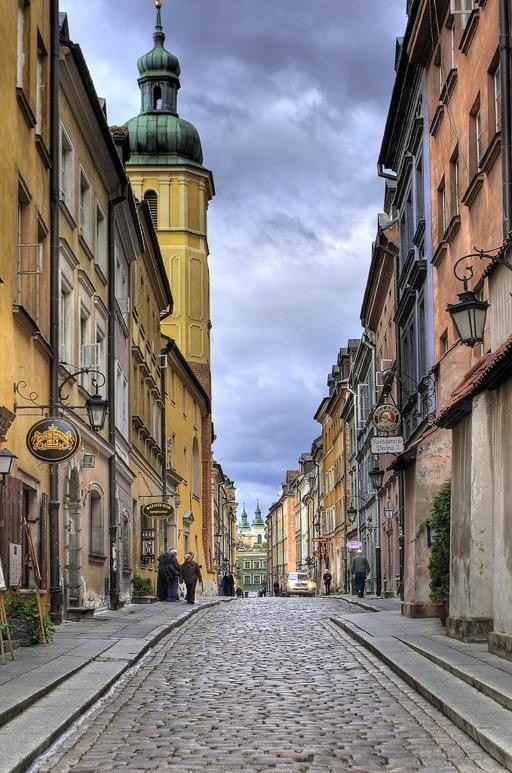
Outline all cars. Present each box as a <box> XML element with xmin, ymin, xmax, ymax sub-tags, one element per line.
<box><xmin>248</xmin><ymin>590</ymin><xmax>257</xmax><ymax>597</ymax></box>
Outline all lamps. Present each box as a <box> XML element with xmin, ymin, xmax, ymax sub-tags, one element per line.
<box><xmin>58</xmin><ymin>361</ymin><xmax>110</xmax><ymax>435</ymax></box>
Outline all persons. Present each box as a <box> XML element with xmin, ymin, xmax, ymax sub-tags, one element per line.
<box><xmin>217</xmin><ymin>571</ymin><xmax>234</xmax><ymax>596</ymax></box>
<box><xmin>351</xmin><ymin>551</ymin><xmax>370</xmax><ymax>597</ymax></box>
<box><xmin>323</xmin><ymin>568</ymin><xmax>331</xmax><ymax>595</ymax></box>
<box><xmin>157</xmin><ymin>548</ymin><xmax>202</xmax><ymax>603</ymax></box>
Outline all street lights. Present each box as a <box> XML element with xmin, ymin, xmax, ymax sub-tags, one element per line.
<box><xmin>47</xmin><ymin>355</ymin><xmax>113</xmax><ymax>624</ymax></box>
<box><xmin>347</xmin><ymin>494</ymin><xmax>376</xmax><ymax>593</ymax></box>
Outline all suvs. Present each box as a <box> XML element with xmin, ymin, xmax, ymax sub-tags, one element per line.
<box><xmin>270</xmin><ymin>568</ymin><xmax>316</xmax><ymax>597</ymax></box>
